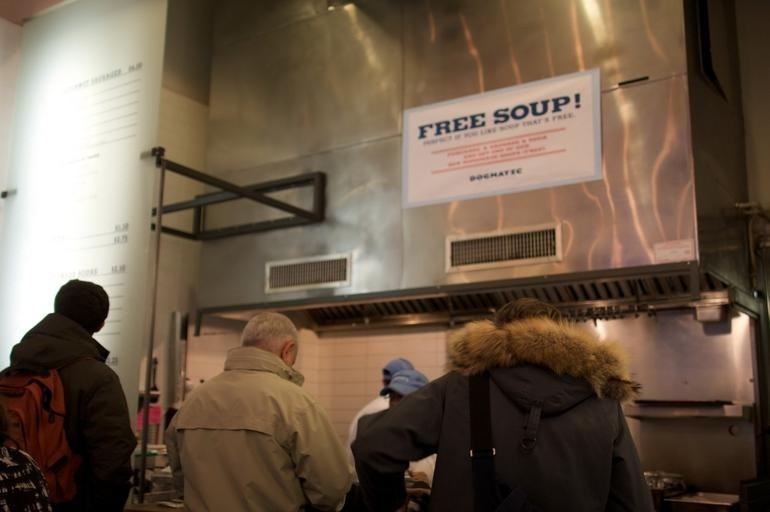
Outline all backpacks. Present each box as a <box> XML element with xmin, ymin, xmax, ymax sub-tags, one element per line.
<box><xmin>1</xmin><ymin>364</ymin><xmax>81</xmax><ymax>511</ymax></box>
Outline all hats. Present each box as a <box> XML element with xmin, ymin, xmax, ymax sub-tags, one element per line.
<box><xmin>380</xmin><ymin>370</ymin><xmax>429</xmax><ymax>397</ymax></box>
<box><xmin>383</xmin><ymin>359</ymin><xmax>414</xmax><ymax>380</ymax></box>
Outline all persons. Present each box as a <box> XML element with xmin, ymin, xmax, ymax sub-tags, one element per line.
<box><xmin>1</xmin><ymin>404</ymin><xmax>53</xmax><ymax>511</ymax></box>
<box><xmin>349</xmin><ymin>357</ymin><xmax>437</xmax><ymax>511</ymax></box>
<box><xmin>349</xmin><ymin>298</ymin><xmax>654</xmax><ymax>512</ymax></box>
<box><xmin>165</xmin><ymin>313</ymin><xmax>354</xmax><ymax>511</ymax></box>
<box><xmin>11</xmin><ymin>281</ymin><xmax>137</xmax><ymax>512</ymax></box>
